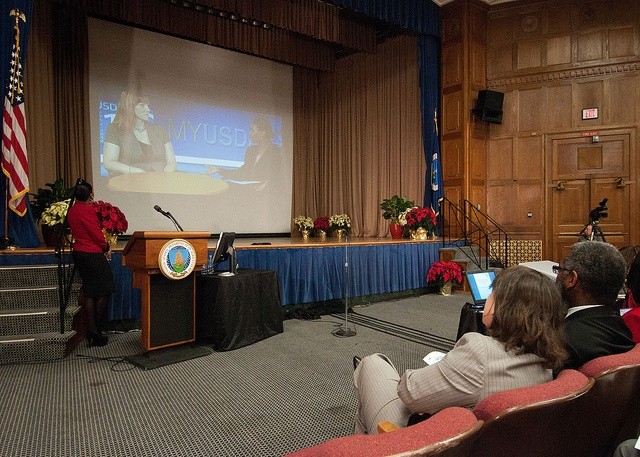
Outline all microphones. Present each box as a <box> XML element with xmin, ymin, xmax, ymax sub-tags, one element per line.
<box><xmin>153</xmin><ymin>205</ymin><xmax>166</xmax><ymax>215</ymax></box>
<box><xmin>343</xmin><ymin>221</ymin><xmax>349</xmax><ymax>234</ymax></box>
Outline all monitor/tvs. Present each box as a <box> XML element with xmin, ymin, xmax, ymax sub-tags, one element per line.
<box><xmin>211</xmin><ymin>231</ymin><xmax>236</xmax><ymax>261</ymax></box>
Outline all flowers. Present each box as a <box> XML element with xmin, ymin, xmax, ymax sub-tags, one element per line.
<box><xmin>404</xmin><ymin>207</ymin><xmax>437</xmax><ymax>231</ymax></box>
<box><xmin>425</xmin><ymin>261</ymin><xmax>463</xmax><ymax>290</ymax></box>
<box><xmin>328</xmin><ymin>214</ymin><xmax>352</xmax><ymax>232</ymax></box>
<box><xmin>89</xmin><ymin>200</ymin><xmax>128</xmax><ymax>236</ymax></box>
<box><xmin>397</xmin><ymin>206</ymin><xmax>418</xmax><ymax>236</ymax></box>
<box><xmin>313</xmin><ymin>217</ymin><xmax>330</xmax><ymax>232</ymax></box>
<box><xmin>293</xmin><ymin>216</ymin><xmax>314</xmax><ymax>233</ymax></box>
<box><xmin>41</xmin><ymin>199</ymin><xmax>74</xmax><ymax>234</ymax></box>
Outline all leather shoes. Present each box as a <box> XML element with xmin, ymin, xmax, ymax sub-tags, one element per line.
<box><xmin>353</xmin><ymin>356</ymin><xmax>361</xmax><ymax>371</ymax></box>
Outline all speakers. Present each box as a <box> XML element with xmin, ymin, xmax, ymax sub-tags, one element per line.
<box><xmin>473</xmin><ymin>88</ymin><xmax>504</xmax><ymax>125</ymax></box>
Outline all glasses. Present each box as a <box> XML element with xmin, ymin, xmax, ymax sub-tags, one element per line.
<box><xmin>552</xmin><ymin>266</ymin><xmax>572</xmax><ymax>274</ymax></box>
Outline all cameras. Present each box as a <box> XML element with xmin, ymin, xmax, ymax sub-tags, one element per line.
<box><xmin>589</xmin><ymin>198</ymin><xmax>608</xmax><ymax>220</ymax></box>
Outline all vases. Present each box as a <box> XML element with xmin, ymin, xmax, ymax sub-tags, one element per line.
<box><xmin>438</xmin><ymin>281</ymin><xmax>452</xmax><ymax>296</ymax></box>
<box><xmin>302</xmin><ymin>230</ymin><xmax>309</xmax><ymax>239</ymax></box>
<box><xmin>414</xmin><ymin>227</ymin><xmax>428</xmax><ymax>241</ymax></box>
<box><xmin>100</xmin><ymin>228</ymin><xmax>118</xmax><ymax>247</ymax></box>
<box><xmin>318</xmin><ymin>229</ymin><xmax>327</xmax><ymax>240</ymax></box>
<box><xmin>335</xmin><ymin>229</ymin><xmax>343</xmax><ymax>239</ymax></box>
<box><xmin>408</xmin><ymin>229</ymin><xmax>414</xmax><ymax>240</ymax></box>
<box><xmin>63</xmin><ymin>231</ymin><xmax>75</xmax><ymax>248</ymax></box>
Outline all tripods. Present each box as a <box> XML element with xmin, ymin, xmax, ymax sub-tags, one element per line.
<box><xmin>587</xmin><ymin>223</ymin><xmax>605</xmax><ymax>242</ymax></box>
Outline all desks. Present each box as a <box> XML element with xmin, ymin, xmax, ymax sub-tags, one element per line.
<box><xmin>196</xmin><ymin>267</ymin><xmax>283</xmax><ymax>352</ymax></box>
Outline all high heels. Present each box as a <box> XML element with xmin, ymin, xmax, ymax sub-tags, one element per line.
<box><xmin>86</xmin><ymin>332</ymin><xmax>108</xmax><ymax>348</ymax></box>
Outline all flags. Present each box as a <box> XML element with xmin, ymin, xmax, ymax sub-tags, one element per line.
<box><xmin>1</xmin><ymin>9</ymin><xmax>30</xmax><ymax>217</ymax></box>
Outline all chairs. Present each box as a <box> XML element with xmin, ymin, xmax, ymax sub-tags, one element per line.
<box><xmin>580</xmin><ymin>343</ymin><xmax>640</xmax><ymax>457</ymax></box>
<box><xmin>471</xmin><ymin>368</ymin><xmax>595</xmax><ymax>457</ymax></box>
<box><xmin>282</xmin><ymin>407</ymin><xmax>485</xmax><ymax>457</ymax></box>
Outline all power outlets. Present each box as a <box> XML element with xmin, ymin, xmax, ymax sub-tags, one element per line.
<box><xmin>527</xmin><ymin>211</ymin><xmax>533</xmax><ymax>217</ymax></box>
<box><xmin>477</xmin><ymin>203</ymin><xmax>480</xmax><ymax>210</ymax></box>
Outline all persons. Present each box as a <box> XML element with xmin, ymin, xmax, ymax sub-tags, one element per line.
<box><xmin>622</xmin><ymin>252</ymin><xmax>640</xmax><ymax>343</ymax></box>
<box><xmin>578</xmin><ymin>225</ymin><xmax>593</xmax><ymax>242</ymax></box>
<box><xmin>102</xmin><ymin>89</ymin><xmax>176</xmax><ymax>177</ymax></box>
<box><xmin>201</xmin><ymin>117</ymin><xmax>289</xmax><ymax>192</ymax></box>
<box><xmin>68</xmin><ymin>181</ymin><xmax>115</xmax><ymax>346</ymax></box>
<box><xmin>354</xmin><ymin>266</ymin><xmax>567</xmax><ymax>438</ymax></box>
<box><xmin>556</xmin><ymin>241</ymin><xmax>635</xmax><ymax>375</ymax></box>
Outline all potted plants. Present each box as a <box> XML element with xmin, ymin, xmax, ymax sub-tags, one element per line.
<box><xmin>379</xmin><ymin>195</ymin><xmax>415</xmax><ymax>240</ymax></box>
<box><xmin>27</xmin><ymin>178</ymin><xmax>76</xmax><ymax>247</ymax></box>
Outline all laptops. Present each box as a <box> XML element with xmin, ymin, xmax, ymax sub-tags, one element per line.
<box><xmin>466</xmin><ymin>269</ymin><xmax>497</xmax><ymax>307</ymax></box>
